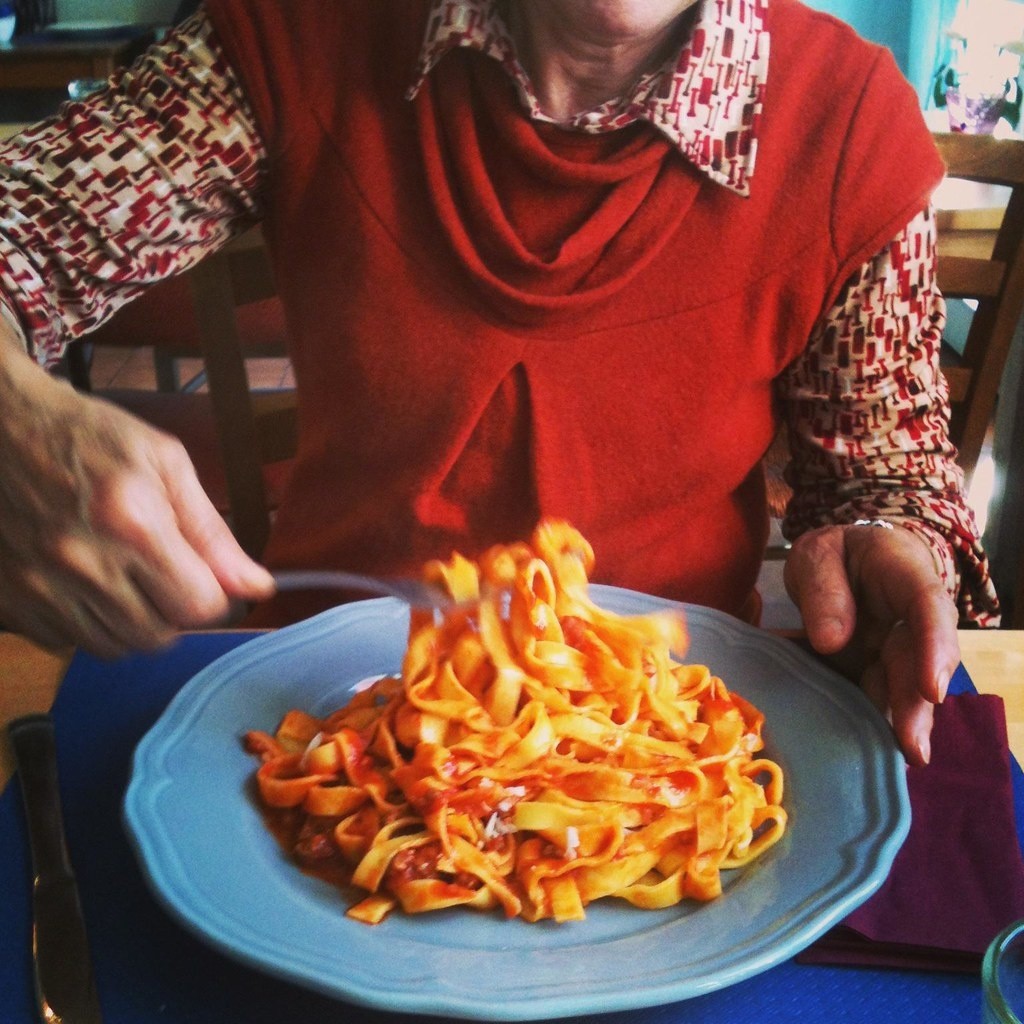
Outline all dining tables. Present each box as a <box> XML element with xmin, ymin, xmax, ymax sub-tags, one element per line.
<box><xmin>0</xmin><ymin>628</ymin><xmax>1024</xmax><ymax>1024</ymax></box>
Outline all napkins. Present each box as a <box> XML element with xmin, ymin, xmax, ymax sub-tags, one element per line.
<box><xmin>796</xmin><ymin>692</ymin><xmax>1024</xmax><ymax>972</ymax></box>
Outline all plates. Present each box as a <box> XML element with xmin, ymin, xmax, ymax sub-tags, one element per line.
<box><xmin>120</xmin><ymin>580</ymin><xmax>913</xmax><ymax>1022</ymax></box>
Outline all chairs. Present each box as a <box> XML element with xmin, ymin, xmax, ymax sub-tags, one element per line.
<box><xmin>767</xmin><ymin>131</ymin><xmax>1024</xmax><ymax>513</ymax></box>
<box><xmin>56</xmin><ymin>224</ymin><xmax>316</xmax><ymax>613</ymax></box>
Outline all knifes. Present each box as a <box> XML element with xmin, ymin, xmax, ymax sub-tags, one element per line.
<box><xmin>5</xmin><ymin>713</ymin><xmax>106</xmax><ymax>1024</ymax></box>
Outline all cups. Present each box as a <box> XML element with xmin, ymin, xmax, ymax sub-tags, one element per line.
<box><xmin>980</xmin><ymin>917</ymin><xmax>1024</xmax><ymax>1024</ymax></box>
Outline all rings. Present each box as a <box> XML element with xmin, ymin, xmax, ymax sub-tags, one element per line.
<box><xmin>886</xmin><ymin>620</ymin><xmax>909</xmax><ymax>638</ymax></box>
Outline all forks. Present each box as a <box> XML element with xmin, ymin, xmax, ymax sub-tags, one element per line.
<box><xmin>274</xmin><ymin>569</ymin><xmax>506</xmax><ymax>611</ymax></box>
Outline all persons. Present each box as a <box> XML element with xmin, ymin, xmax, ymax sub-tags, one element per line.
<box><xmin>0</xmin><ymin>0</ymin><xmax>1008</xmax><ymax>771</ymax></box>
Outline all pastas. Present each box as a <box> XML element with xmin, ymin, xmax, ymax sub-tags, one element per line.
<box><xmin>245</xmin><ymin>518</ymin><xmax>788</xmax><ymax>926</ymax></box>
